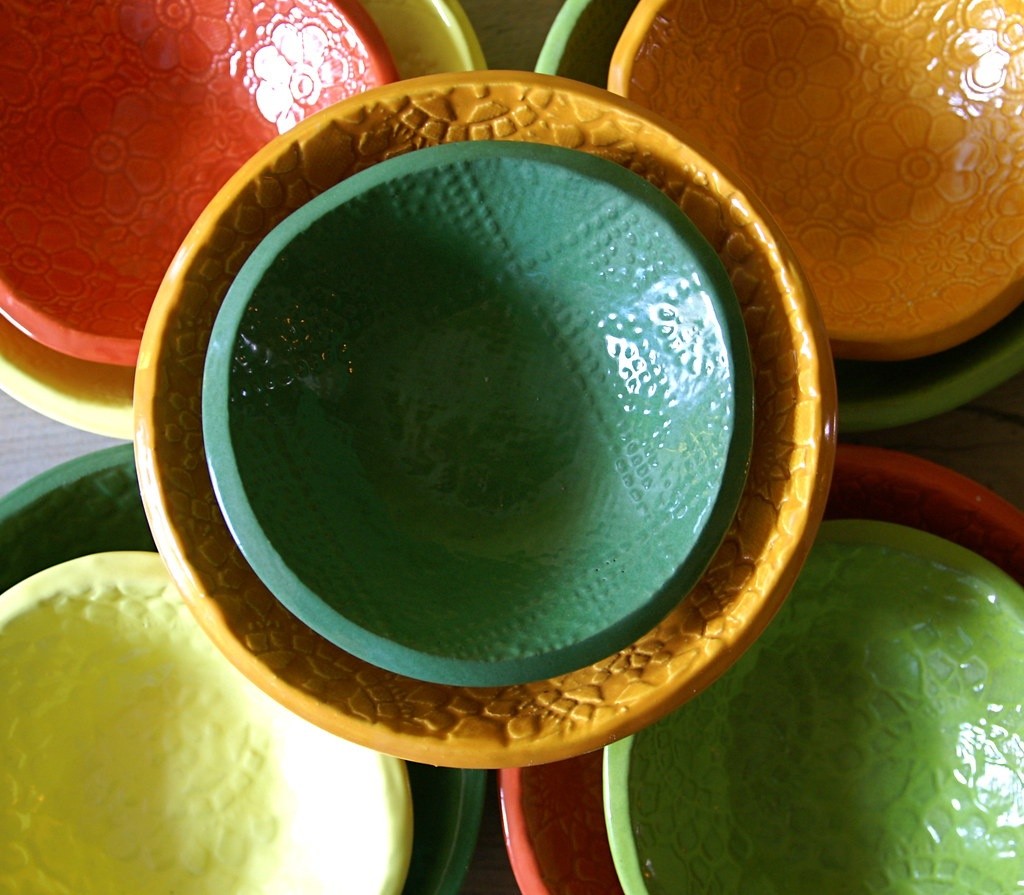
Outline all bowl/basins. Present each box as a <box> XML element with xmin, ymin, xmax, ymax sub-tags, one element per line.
<box><xmin>4</xmin><ymin>0</ymin><xmax>1024</xmax><ymax>895</ymax></box>
